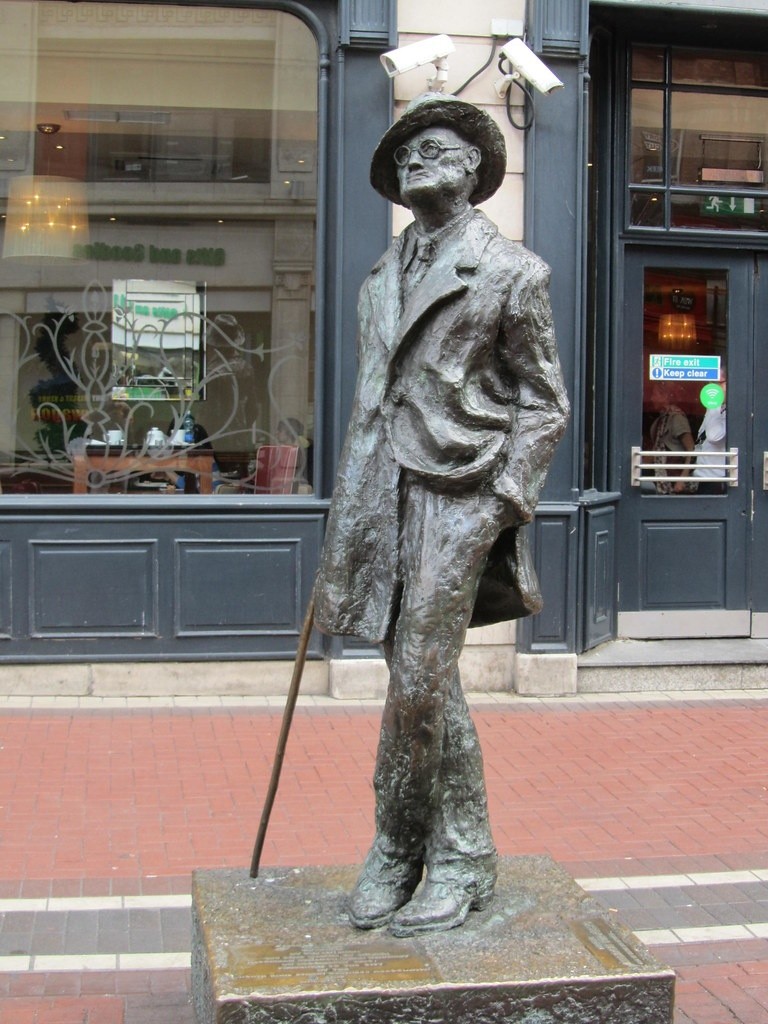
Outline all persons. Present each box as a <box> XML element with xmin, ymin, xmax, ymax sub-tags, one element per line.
<box><xmin>144</xmin><ymin>413</ymin><xmax>221</xmax><ymax>494</ymax></box>
<box><xmin>313</xmin><ymin>92</ymin><xmax>572</xmax><ymax>937</ymax></box>
<box><xmin>642</xmin><ymin>366</ymin><xmax>727</xmax><ymax>495</ymax></box>
<box><xmin>225</xmin><ymin>418</ymin><xmax>306</xmax><ymax>494</ymax></box>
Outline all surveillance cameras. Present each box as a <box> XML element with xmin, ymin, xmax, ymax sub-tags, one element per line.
<box><xmin>503</xmin><ymin>38</ymin><xmax>564</xmax><ymax>96</ymax></box>
<box><xmin>379</xmin><ymin>34</ymin><xmax>455</xmax><ymax>77</ymax></box>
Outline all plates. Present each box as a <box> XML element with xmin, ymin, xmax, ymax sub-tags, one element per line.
<box><xmin>135</xmin><ymin>482</ymin><xmax>167</xmax><ymax>489</ymax></box>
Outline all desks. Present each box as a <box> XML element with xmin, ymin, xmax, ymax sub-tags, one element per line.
<box><xmin>73</xmin><ymin>447</ymin><xmax>216</xmax><ymax>494</ymax></box>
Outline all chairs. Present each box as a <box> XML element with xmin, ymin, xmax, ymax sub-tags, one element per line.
<box><xmin>254</xmin><ymin>445</ymin><xmax>300</xmax><ymax>494</ymax></box>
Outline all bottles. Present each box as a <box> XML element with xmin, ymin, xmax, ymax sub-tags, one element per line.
<box><xmin>184</xmin><ymin>410</ymin><xmax>195</xmax><ymax>443</ymax></box>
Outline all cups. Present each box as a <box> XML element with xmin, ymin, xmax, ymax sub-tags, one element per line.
<box><xmin>103</xmin><ymin>430</ymin><xmax>122</xmax><ymax>445</ymax></box>
<box><xmin>170</xmin><ymin>430</ymin><xmax>185</xmax><ymax>443</ymax></box>
<box><xmin>146</xmin><ymin>427</ymin><xmax>165</xmax><ymax>445</ymax></box>
